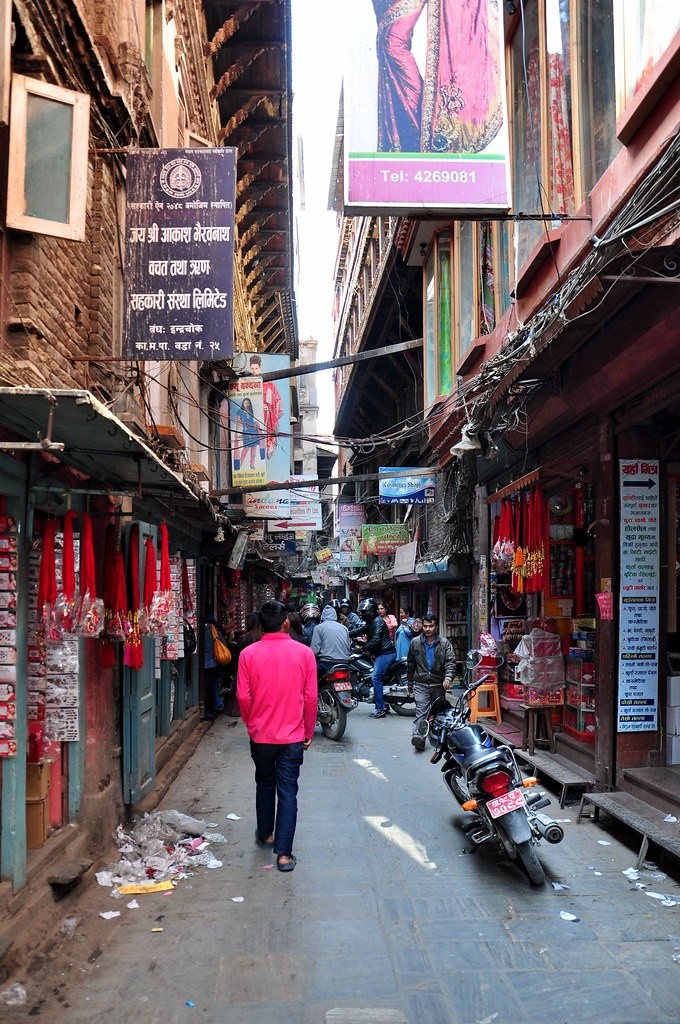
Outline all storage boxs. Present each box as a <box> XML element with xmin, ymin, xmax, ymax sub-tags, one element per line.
<box><xmin>520</xmin><ymin>635</ymin><xmax>564</xmax><ymax>706</ymax></box>
<box><xmin>26</xmin><ymin>759</ymin><xmax>51</xmax><ymax>801</ymax></box>
<box><xmin>26</xmin><ymin>797</ymin><xmax>49</xmax><ymax>850</ymax></box>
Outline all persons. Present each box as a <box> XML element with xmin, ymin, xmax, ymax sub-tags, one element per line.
<box><xmin>234</xmin><ymin>397</ymin><xmax>260</xmax><ymax>469</ymax></box>
<box><xmin>371</xmin><ymin>0</ymin><xmax>429</xmax><ymax>152</ymax></box>
<box><xmin>248</xmin><ymin>354</ymin><xmax>282</xmax><ymax>459</ymax></box>
<box><xmin>406</xmin><ymin>614</ymin><xmax>457</xmax><ymax>751</ymax></box>
<box><xmin>203</xmin><ymin>596</ymin><xmax>421</xmax><ymax>720</ymax></box>
<box><xmin>343</xmin><ymin>539</ymin><xmax>353</xmax><ymax>550</ymax></box>
<box><xmin>235</xmin><ymin>599</ymin><xmax>319</xmax><ymax>872</ymax></box>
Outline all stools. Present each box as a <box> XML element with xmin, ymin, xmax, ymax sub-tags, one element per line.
<box><xmin>466</xmin><ymin>684</ymin><xmax>502</xmax><ymax>726</ymax></box>
<box><xmin>518</xmin><ymin>704</ymin><xmax>557</xmax><ymax>756</ymax></box>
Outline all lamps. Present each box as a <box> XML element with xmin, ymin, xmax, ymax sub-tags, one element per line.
<box><xmin>191</xmin><ymin>464</ymin><xmax>211</xmax><ymax>481</ymax></box>
<box><xmin>149</xmin><ymin>424</ymin><xmax>186</xmax><ymax>450</ymax></box>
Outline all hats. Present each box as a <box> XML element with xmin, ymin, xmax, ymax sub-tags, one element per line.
<box><xmin>341</xmin><ymin>598</ymin><xmax>350</xmax><ymax>607</ymax></box>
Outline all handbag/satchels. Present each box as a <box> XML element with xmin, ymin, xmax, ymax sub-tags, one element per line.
<box><xmin>409</xmin><ymin>618</ymin><xmax>423</xmax><ymax>637</ymax></box>
<box><xmin>211</xmin><ymin>624</ymin><xmax>231</xmax><ymax>666</ymax></box>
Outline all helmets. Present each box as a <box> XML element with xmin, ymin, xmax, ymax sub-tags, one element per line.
<box><xmin>359</xmin><ymin>599</ymin><xmax>378</xmax><ymax>621</ymax></box>
<box><xmin>299</xmin><ymin>603</ymin><xmax>321</xmax><ymax>625</ymax></box>
<box><xmin>328</xmin><ymin>599</ymin><xmax>342</xmax><ymax>610</ymax></box>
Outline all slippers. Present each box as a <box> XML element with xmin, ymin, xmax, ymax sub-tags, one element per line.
<box><xmin>278</xmin><ymin>853</ymin><xmax>296</xmax><ymax>871</ymax></box>
<box><xmin>255</xmin><ymin>831</ymin><xmax>273</xmax><ymax>847</ymax></box>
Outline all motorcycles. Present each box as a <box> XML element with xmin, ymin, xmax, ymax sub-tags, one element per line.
<box><xmin>303</xmin><ymin>664</ymin><xmax>359</xmax><ymax>742</ymax></box>
<box><xmin>347</xmin><ymin>637</ymin><xmax>417</xmax><ymax>717</ymax></box>
<box><xmin>415</xmin><ymin>650</ymin><xmax>565</xmax><ymax>886</ymax></box>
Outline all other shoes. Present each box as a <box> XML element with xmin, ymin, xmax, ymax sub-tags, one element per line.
<box><xmin>367</xmin><ymin>709</ymin><xmax>385</xmax><ymax>719</ymax></box>
<box><xmin>412</xmin><ymin>735</ymin><xmax>424</xmax><ymax>752</ymax></box>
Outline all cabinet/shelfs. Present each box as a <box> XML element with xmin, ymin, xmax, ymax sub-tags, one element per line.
<box><xmin>561</xmin><ymin>646</ymin><xmax>595</xmax><ymax>742</ymax></box>
<box><xmin>438</xmin><ymin>585</ymin><xmax>467</xmax><ymax>666</ymax></box>
